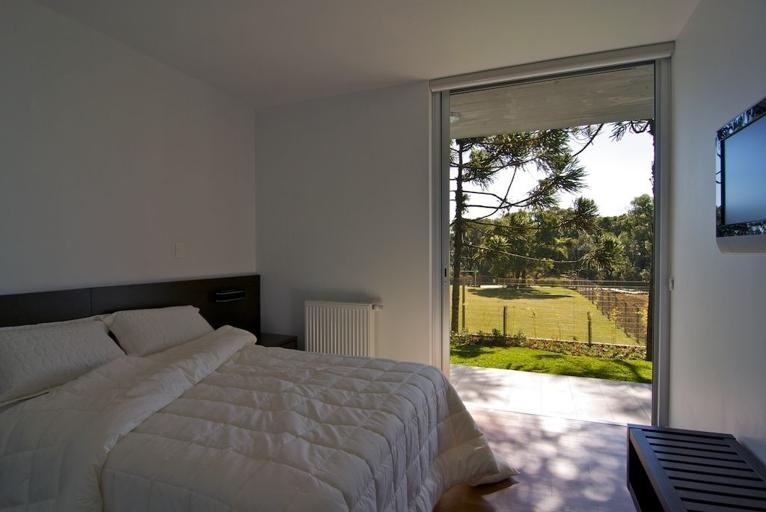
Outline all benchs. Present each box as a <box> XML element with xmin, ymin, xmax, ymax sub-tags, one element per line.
<box><xmin>624</xmin><ymin>416</ymin><xmax>766</xmax><ymax>511</ymax></box>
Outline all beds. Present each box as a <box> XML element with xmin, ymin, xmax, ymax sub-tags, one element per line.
<box><xmin>0</xmin><ymin>274</ymin><xmax>520</xmax><ymax>512</ymax></box>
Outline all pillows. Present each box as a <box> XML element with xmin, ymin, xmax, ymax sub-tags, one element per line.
<box><xmin>0</xmin><ymin>311</ymin><xmax>129</xmax><ymax>407</ymax></box>
<box><xmin>99</xmin><ymin>304</ymin><xmax>216</xmax><ymax>356</ymax></box>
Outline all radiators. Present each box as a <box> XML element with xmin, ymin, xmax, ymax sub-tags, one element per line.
<box><xmin>303</xmin><ymin>299</ymin><xmax>383</xmax><ymax>357</ymax></box>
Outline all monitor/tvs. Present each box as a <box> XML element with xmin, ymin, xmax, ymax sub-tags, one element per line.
<box><xmin>715</xmin><ymin>98</ymin><xmax>766</xmax><ymax>237</ymax></box>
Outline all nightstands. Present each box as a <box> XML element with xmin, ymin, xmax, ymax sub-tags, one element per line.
<box><xmin>244</xmin><ymin>327</ymin><xmax>299</xmax><ymax>350</ymax></box>
